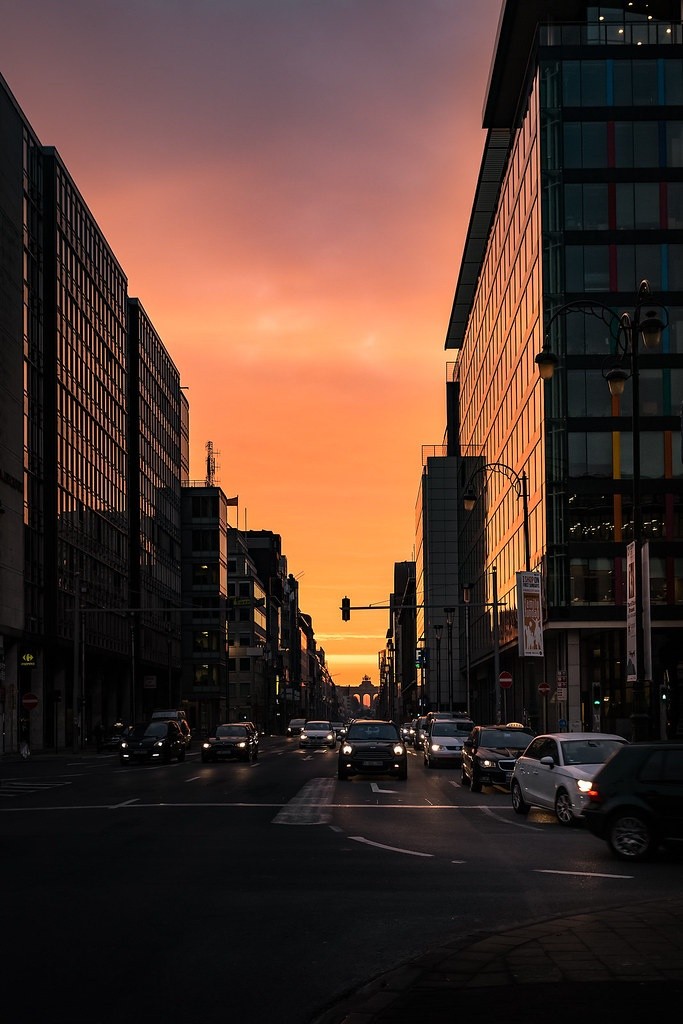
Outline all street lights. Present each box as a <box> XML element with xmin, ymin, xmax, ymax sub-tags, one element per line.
<box><xmin>433</xmin><ymin>624</ymin><xmax>444</xmax><ymax>710</ymax></box>
<box><xmin>292</xmin><ymin>681</ymin><xmax>306</xmax><ymax>718</ymax></box>
<box><xmin>325</xmin><ymin>700</ymin><xmax>332</xmax><ymax>721</ymax></box>
<box><xmin>418</xmin><ymin>638</ymin><xmax>427</xmax><ymax>716</ymax></box>
<box><xmin>368</xmin><ymin>640</ymin><xmax>395</xmax><ymax>720</ymax></box>
<box><xmin>462</xmin><ymin>583</ymin><xmax>474</xmax><ymax>717</ymax></box>
<box><xmin>444</xmin><ymin>608</ymin><xmax>455</xmax><ymax>711</ymax></box>
<box><xmin>533</xmin><ymin>280</ymin><xmax>670</xmax><ymax>733</ymax></box>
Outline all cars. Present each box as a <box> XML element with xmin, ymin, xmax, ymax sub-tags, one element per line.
<box><xmin>336</xmin><ymin>720</ymin><xmax>411</xmax><ymax>781</ymax></box>
<box><xmin>582</xmin><ymin>745</ymin><xmax>682</xmax><ymax>866</ymax></box>
<box><xmin>459</xmin><ymin>727</ymin><xmax>539</xmax><ymax>792</ymax></box>
<box><xmin>423</xmin><ymin>719</ymin><xmax>477</xmax><ymax>767</ymax></box>
<box><xmin>287</xmin><ymin>719</ymin><xmax>308</xmax><ymax>736</ymax></box>
<box><xmin>298</xmin><ymin>721</ymin><xmax>337</xmax><ymax>748</ymax></box>
<box><xmin>510</xmin><ymin>732</ymin><xmax>632</xmax><ymax>826</ymax></box>
<box><xmin>332</xmin><ymin>722</ymin><xmax>350</xmax><ymax>737</ymax></box>
<box><xmin>400</xmin><ymin>711</ymin><xmax>472</xmax><ymax>748</ymax></box>
<box><xmin>120</xmin><ymin>710</ymin><xmax>192</xmax><ymax>765</ymax></box>
<box><xmin>200</xmin><ymin>720</ymin><xmax>260</xmax><ymax>762</ymax></box>
<box><xmin>96</xmin><ymin>722</ymin><xmax>145</xmax><ymax>754</ymax></box>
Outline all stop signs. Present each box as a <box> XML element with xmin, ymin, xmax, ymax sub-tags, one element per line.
<box><xmin>498</xmin><ymin>671</ymin><xmax>513</xmax><ymax>689</ymax></box>
<box><xmin>22</xmin><ymin>693</ymin><xmax>38</xmax><ymax>711</ymax></box>
<box><xmin>539</xmin><ymin>683</ymin><xmax>551</xmax><ymax>696</ymax></box>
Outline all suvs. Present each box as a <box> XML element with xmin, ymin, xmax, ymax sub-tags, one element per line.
<box><xmin>364</xmin><ymin>727</ymin><xmax>380</xmax><ymax>736</ymax></box>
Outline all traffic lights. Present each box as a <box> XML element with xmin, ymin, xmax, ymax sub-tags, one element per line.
<box><xmin>415</xmin><ymin>663</ymin><xmax>422</xmax><ymax>668</ymax></box>
